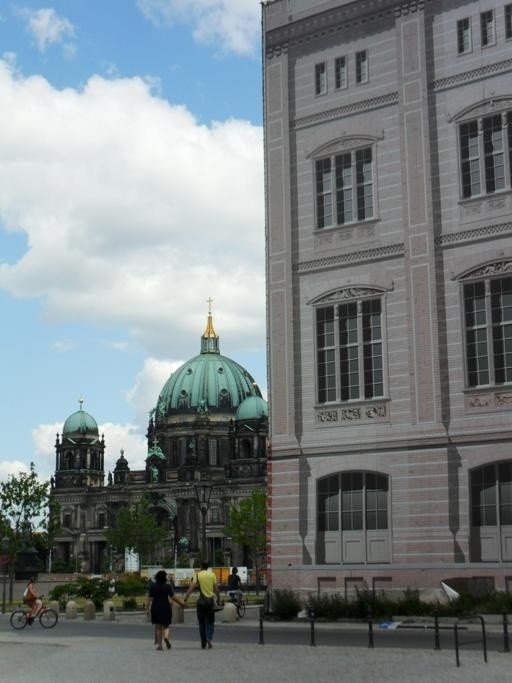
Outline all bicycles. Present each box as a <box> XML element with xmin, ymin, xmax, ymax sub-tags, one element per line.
<box><xmin>10</xmin><ymin>595</ymin><xmax>58</xmax><ymax>629</ymax></box>
<box><xmin>229</xmin><ymin>586</ymin><xmax>245</xmax><ymax>617</ymax></box>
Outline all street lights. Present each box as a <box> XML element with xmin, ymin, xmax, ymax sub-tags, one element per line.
<box><xmin>194</xmin><ymin>486</ymin><xmax>213</xmax><ymax>561</ymax></box>
<box><xmin>248</xmin><ymin>535</ymin><xmax>259</xmax><ymax>595</ymax></box>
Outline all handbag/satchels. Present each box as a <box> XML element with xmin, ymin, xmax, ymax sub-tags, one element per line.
<box><xmin>199</xmin><ymin>594</ymin><xmax>218</xmax><ymax>610</ymax></box>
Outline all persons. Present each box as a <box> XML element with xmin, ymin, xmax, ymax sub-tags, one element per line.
<box><xmin>149</xmin><ymin>600</ymin><xmax>173</xmax><ymax>644</ymax></box>
<box><xmin>228</xmin><ymin>565</ymin><xmax>245</xmax><ymax>608</ymax></box>
<box><xmin>184</xmin><ymin>562</ymin><xmax>223</xmax><ymax>647</ymax></box>
<box><xmin>144</xmin><ymin>567</ymin><xmax>186</xmax><ymax>653</ymax></box>
<box><xmin>24</xmin><ymin>574</ymin><xmax>44</xmax><ymax>626</ymax></box>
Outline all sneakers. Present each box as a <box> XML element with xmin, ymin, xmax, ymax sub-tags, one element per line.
<box><xmin>164</xmin><ymin>637</ymin><xmax>171</xmax><ymax>650</ymax></box>
<box><xmin>153</xmin><ymin>639</ymin><xmax>163</xmax><ymax>651</ymax></box>
<box><xmin>207</xmin><ymin>638</ymin><xmax>214</xmax><ymax>648</ymax></box>
